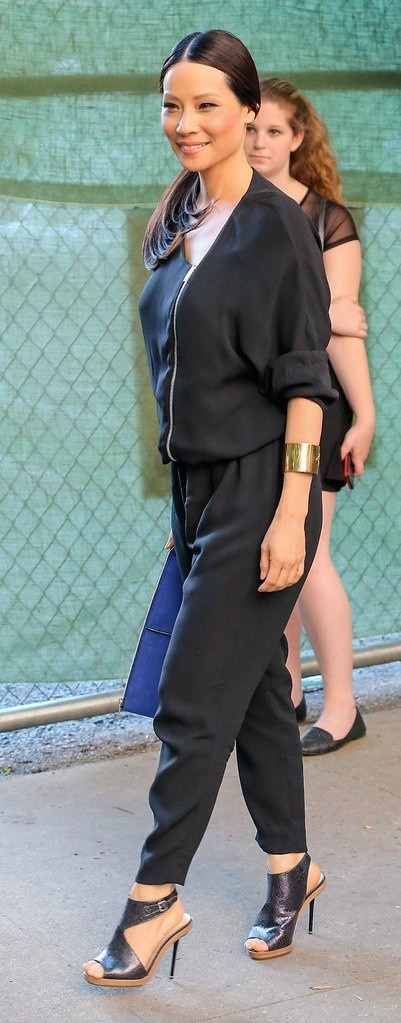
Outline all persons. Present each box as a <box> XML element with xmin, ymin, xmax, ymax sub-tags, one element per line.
<box><xmin>246</xmin><ymin>76</ymin><xmax>375</xmax><ymax>756</ymax></box>
<box><xmin>82</xmin><ymin>30</ymin><xmax>339</xmax><ymax>986</ymax></box>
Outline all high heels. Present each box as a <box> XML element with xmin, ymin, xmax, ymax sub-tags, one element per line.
<box><xmin>246</xmin><ymin>853</ymin><xmax>328</xmax><ymax>961</ymax></box>
<box><xmin>82</xmin><ymin>887</ymin><xmax>193</xmax><ymax>988</ymax></box>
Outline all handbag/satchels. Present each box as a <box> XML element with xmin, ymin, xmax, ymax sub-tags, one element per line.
<box><xmin>118</xmin><ymin>546</ymin><xmax>187</xmax><ymax>720</ymax></box>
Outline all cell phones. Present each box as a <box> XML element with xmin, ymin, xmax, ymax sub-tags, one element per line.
<box><xmin>344</xmin><ymin>452</ymin><xmax>355</xmax><ymax>490</ymax></box>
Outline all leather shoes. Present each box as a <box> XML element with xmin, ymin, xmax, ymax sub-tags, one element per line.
<box><xmin>294</xmin><ymin>696</ymin><xmax>308</xmax><ymax>723</ymax></box>
<box><xmin>300</xmin><ymin>706</ymin><xmax>367</xmax><ymax>756</ymax></box>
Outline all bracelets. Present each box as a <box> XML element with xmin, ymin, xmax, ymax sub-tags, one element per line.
<box><xmin>283</xmin><ymin>443</ymin><xmax>320</xmax><ymax>475</ymax></box>
